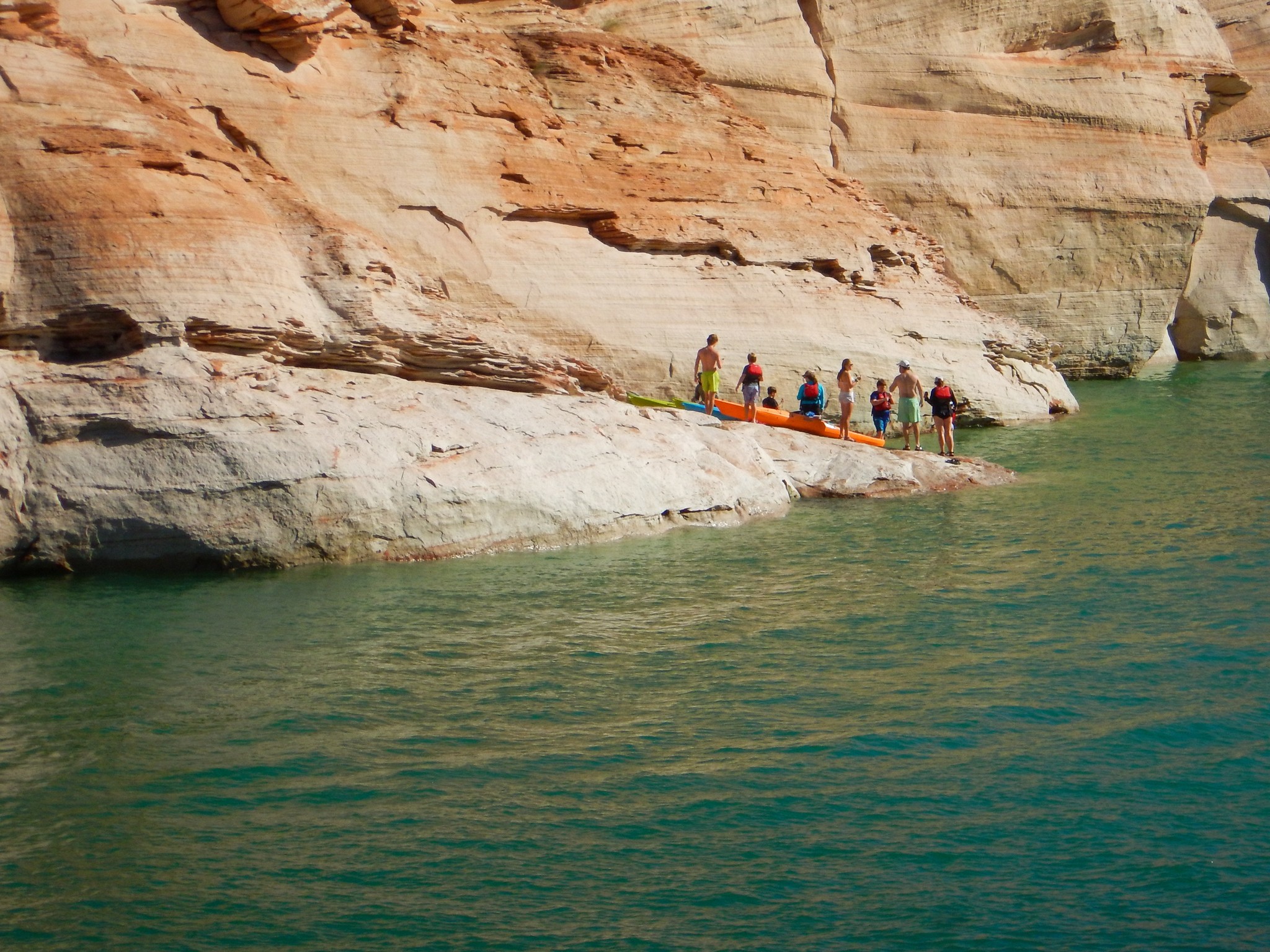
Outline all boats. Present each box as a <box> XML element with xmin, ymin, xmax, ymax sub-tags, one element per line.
<box><xmin>681</xmin><ymin>401</ymin><xmax>737</xmax><ymax>421</ymax></box>
<box><xmin>672</xmin><ymin>397</ymin><xmax>699</xmax><ymax>411</ymax></box>
<box><xmin>713</xmin><ymin>397</ymin><xmax>886</xmax><ymax>448</ymax></box>
<box><xmin>625</xmin><ymin>390</ymin><xmax>676</xmax><ymax>407</ymax></box>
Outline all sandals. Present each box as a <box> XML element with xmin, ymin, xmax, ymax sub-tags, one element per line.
<box><xmin>903</xmin><ymin>445</ymin><xmax>910</xmax><ymax>450</ymax></box>
<box><xmin>945</xmin><ymin>451</ymin><xmax>954</xmax><ymax>456</ymax></box>
<box><xmin>938</xmin><ymin>452</ymin><xmax>945</xmax><ymax>456</ymax></box>
<box><xmin>914</xmin><ymin>446</ymin><xmax>924</xmax><ymax>451</ymax></box>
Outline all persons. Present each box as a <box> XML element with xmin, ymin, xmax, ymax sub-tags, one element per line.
<box><xmin>837</xmin><ymin>358</ymin><xmax>862</xmax><ymax>442</ymax></box>
<box><xmin>889</xmin><ymin>360</ymin><xmax>924</xmax><ymax>451</ymax></box>
<box><xmin>924</xmin><ymin>376</ymin><xmax>958</xmax><ymax>456</ymax></box>
<box><xmin>797</xmin><ymin>370</ymin><xmax>824</xmax><ymax>417</ymax></box>
<box><xmin>691</xmin><ymin>372</ymin><xmax>719</xmax><ymax>407</ymax></box>
<box><xmin>735</xmin><ymin>352</ymin><xmax>763</xmax><ymax>423</ymax></box>
<box><xmin>762</xmin><ymin>386</ymin><xmax>784</xmax><ymax>411</ymax></box>
<box><xmin>870</xmin><ymin>378</ymin><xmax>895</xmax><ymax>440</ymax></box>
<box><xmin>694</xmin><ymin>334</ymin><xmax>722</xmax><ymax>416</ymax></box>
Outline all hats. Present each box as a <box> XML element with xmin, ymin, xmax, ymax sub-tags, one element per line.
<box><xmin>897</xmin><ymin>360</ymin><xmax>910</xmax><ymax>367</ymax></box>
<box><xmin>935</xmin><ymin>376</ymin><xmax>943</xmax><ymax>382</ymax></box>
<box><xmin>802</xmin><ymin>370</ymin><xmax>815</xmax><ymax>376</ymax></box>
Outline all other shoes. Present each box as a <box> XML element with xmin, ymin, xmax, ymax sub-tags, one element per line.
<box><xmin>844</xmin><ymin>436</ymin><xmax>855</xmax><ymax>442</ymax></box>
<box><xmin>839</xmin><ymin>436</ymin><xmax>844</xmax><ymax>440</ymax></box>
